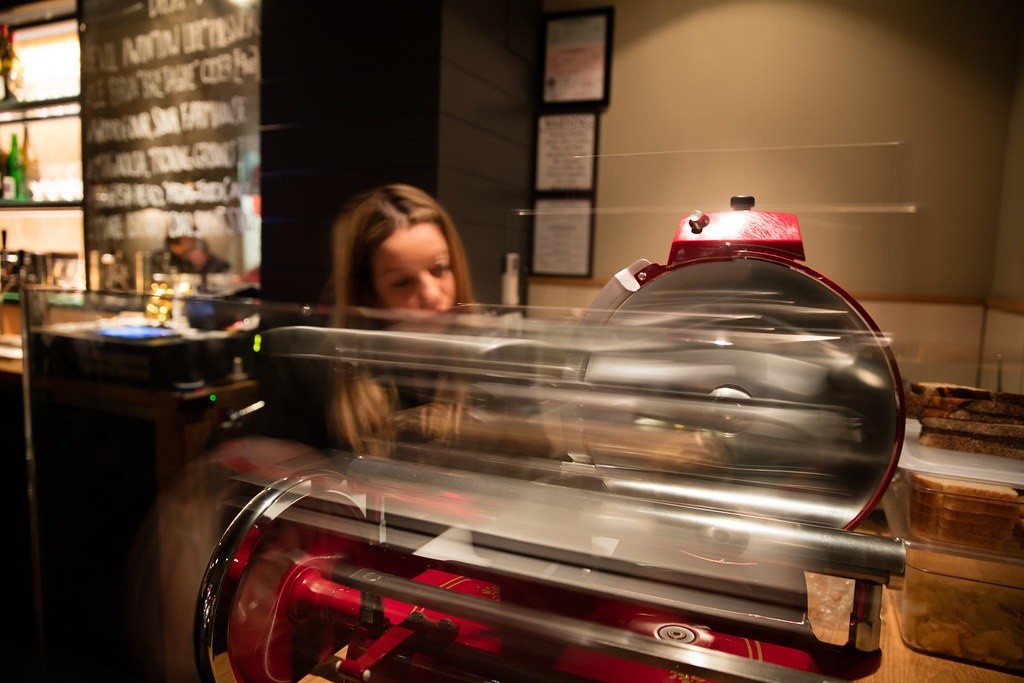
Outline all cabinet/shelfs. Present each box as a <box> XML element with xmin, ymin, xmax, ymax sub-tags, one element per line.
<box><xmin>0</xmin><ymin>12</ymin><xmax>89</xmax><ymax>312</ymax></box>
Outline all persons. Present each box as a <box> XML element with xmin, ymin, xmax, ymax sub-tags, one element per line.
<box><xmin>153</xmin><ymin>221</ymin><xmax>231</xmax><ymax>288</ymax></box>
<box><xmin>290</xmin><ymin>186</ymin><xmax>553</xmax><ymax>459</ymax></box>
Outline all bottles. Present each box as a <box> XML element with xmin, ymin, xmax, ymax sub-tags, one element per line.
<box><xmin>7</xmin><ymin>134</ymin><xmax>28</xmax><ymax>200</ymax></box>
<box><xmin>3</xmin><ymin>230</ymin><xmax>43</xmax><ymax>294</ymax></box>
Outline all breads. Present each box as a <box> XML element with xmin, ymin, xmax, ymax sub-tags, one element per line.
<box><xmin>904</xmin><ymin>471</ymin><xmax>1020</xmax><ymax>552</ymax></box>
<box><xmin>909</xmin><ymin>381</ymin><xmax>1024</xmax><ymax>459</ymax></box>
